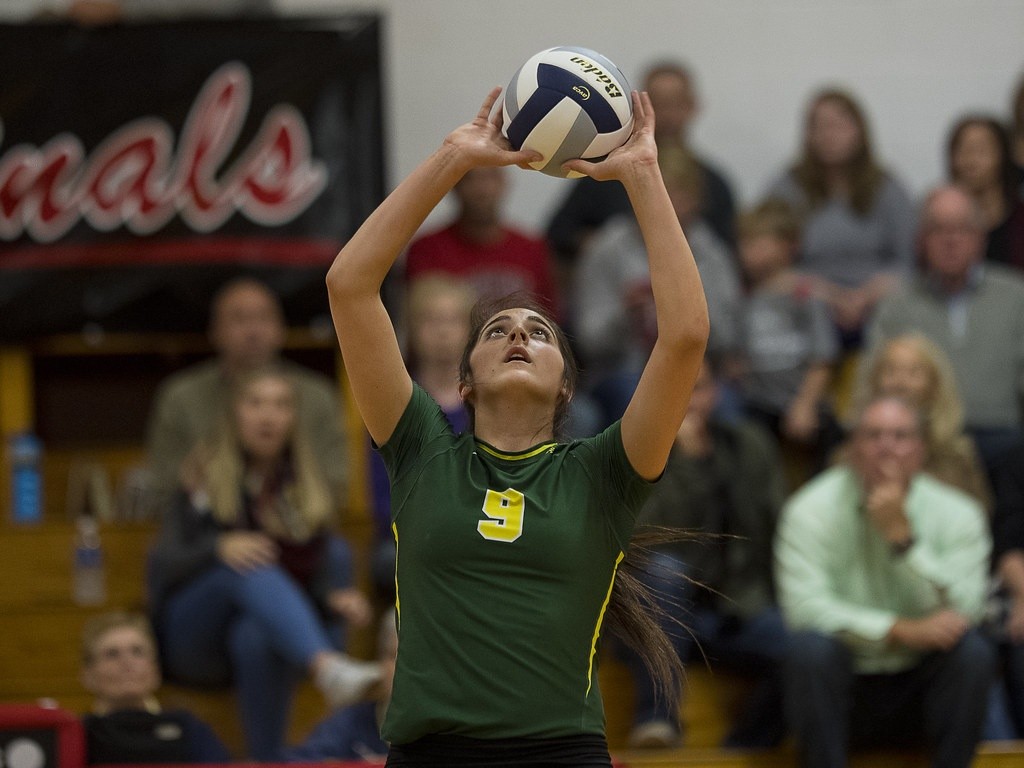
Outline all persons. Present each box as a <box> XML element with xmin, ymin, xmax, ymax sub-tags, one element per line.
<box><xmin>325</xmin><ymin>88</ymin><xmax>712</xmax><ymax>768</ymax></box>
<box><xmin>80</xmin><ymin>62</ymin><xmax>1024</xmax><ymax>768</ymax></box>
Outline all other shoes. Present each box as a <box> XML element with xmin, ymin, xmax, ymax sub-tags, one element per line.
<box><xmin>627</xmin><ymin>720</ymin><xmax>677</xmax><ymax>748</ymax></box>
<box><xmin>313</xmin><ymin>657</ymin><xmax>386</xmax><ymax>710</ymax></box>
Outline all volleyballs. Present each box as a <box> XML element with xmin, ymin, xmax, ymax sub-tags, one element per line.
<box><xmin>503</xmin><ymin>45</ymin><xmax>636</xmax><ymax>179</ymax></box>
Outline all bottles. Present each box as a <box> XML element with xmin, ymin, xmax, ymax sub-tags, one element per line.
<box><xmin>72</xmin><ymin>516</ymin><xmax>104</xmax><ymax>608</ymax></box>
<box><xmin>12</xmin><ymin>435</ymin><xmax>44</xmax><ymax>521</ymax></box>
